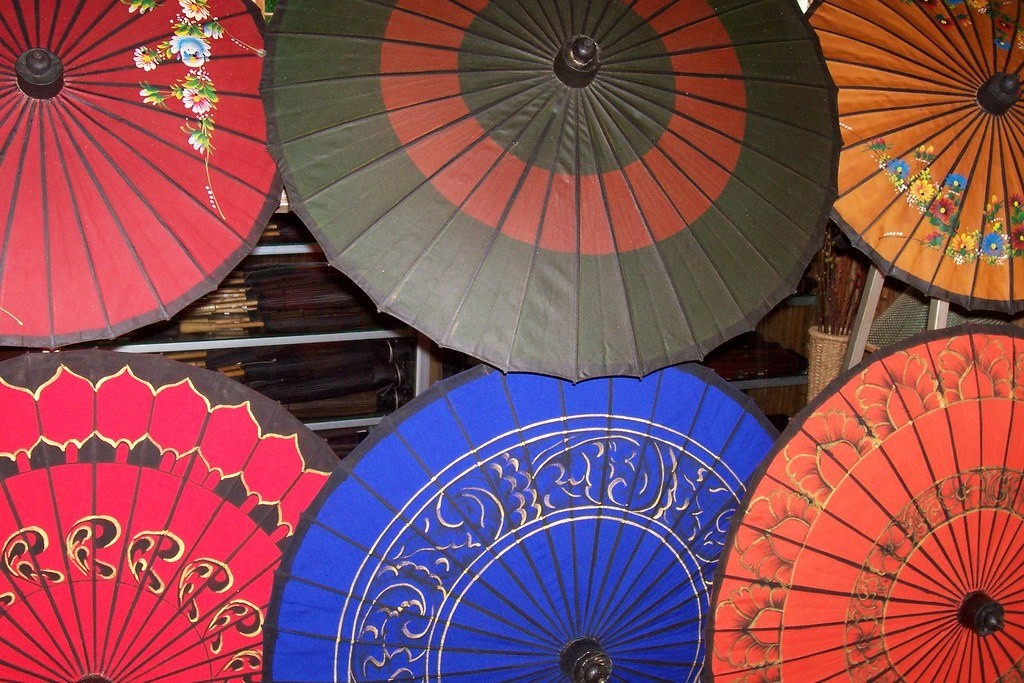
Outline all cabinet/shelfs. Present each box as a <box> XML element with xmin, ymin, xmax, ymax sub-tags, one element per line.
<box><xmin>94</xmin><ymin>244</ymin><xmax>819</xmax><ymax>431</ymax></box>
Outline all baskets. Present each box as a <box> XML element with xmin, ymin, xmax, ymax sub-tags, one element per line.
<box><xmin>806</xmin><ymin>324</ymin><xmax>852</xmax><ymax>405</ymax></box>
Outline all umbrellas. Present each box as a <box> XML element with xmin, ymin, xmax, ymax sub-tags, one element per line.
<box><xmin>704</xmin><ymin>325</ymin><xmax>1024</xmax><ymax>683</ymax></box>
<box><xmin>263</xmin><ymin>360</ymin><xmax>782</xmax><ymax>683</ymax></box>
<box><xmin>804</xmin><ymin>1</ymin><xmax>1024</xmax><ymax>318</ymax></box>
<box><xmin>0</xmin><ymin>353</ymin><xmax>341</xmax><ymax>683</ymax></box>
<box><xmin>1</xmin><ymin>0</ymin><xmax>282</xmax><ymax>352</ymax></box>
<box><xmin>260</xmin><ymin>0</ymin><xmax>843</xmax><ymax>386</ymax></box>
